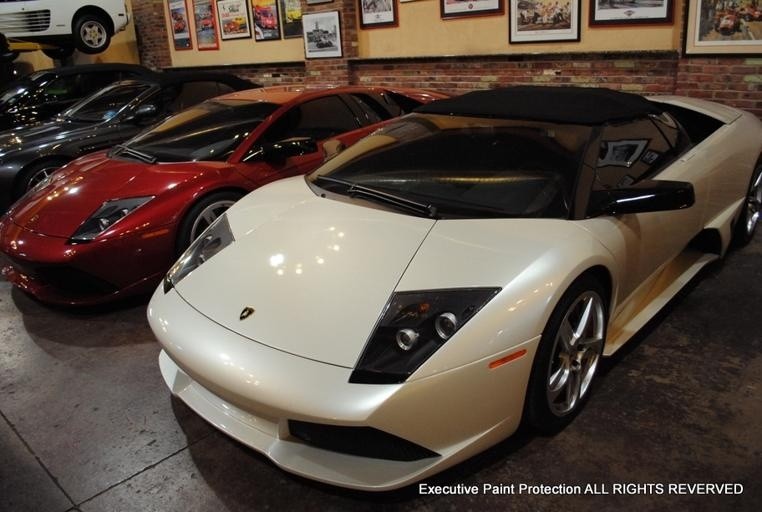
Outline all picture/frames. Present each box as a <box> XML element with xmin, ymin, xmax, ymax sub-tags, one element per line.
<box><xmin>301</xmin><ymin>0</ymin><xmax>345</xmax><ymax>60</ymax></box>
<box><xmin>508</xmin><ymin>0</ymin><xmax>583</xmax><ymax>45</ymax></box>
<box><xmin>166</xmin><ymin>0</ymin><xmax>193</xmax><ymax>52</ymax></box>
<box><xmin>216</xmin><ymin>0</ymin><xmax>252</xmax><ymax>41</ymax></box>
<box><xmin>251</xmin><ymin>0</ymin><xmax>282</xmax><ymax>43</ymax></box>
<box><xmin>439</xmin><ymin>0</ymin><xmax>506</xmax><ymax>20</ymax></box>
<box><xmin>192</xmin><ymin>0</ymin><xmax>220</xmax><ymax>51</ymax></box>
<box><xmin>357</xmin><ymin>0</ymin><xmax>399</xmax><ymax>30</ymax></box>
<box><xmin>279</xmin><ymin>0</ymin><xmax>308</xmax><ymax>40</ymax></box>
<box><xmin>588</xmin><ymin>0</ymin><xmax>762</xmax><ymax>60</ymax></box>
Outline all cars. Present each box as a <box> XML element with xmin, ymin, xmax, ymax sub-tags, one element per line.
<box><xmin>536</xmin><ymin>13</ymin><xmax>554</xmax><ymax>25</ymax></box>
<box><xmin>224</xmin><ymin>21</ymin><xmax>239</xmax><ymax>32</ymax></box>
<box><xmin>287</xmin><ymin>8</ymin><xmax>301</xmax><ymax>20</ymax></box>
<box><xmin>255</xmin><ymin>10</ymin><xmax>275</xmax><ymax>29</ymax></box>
<box><xmin>1</xmin><ymin>74</ymin><xmax>264</xmax><ymax>211</ymax></box>
<box><xmin>0</xmin><ymin>0</ymin><xmax>131</xmax><ymax>65</ymax></box>
<box><xmin>172</xmin><ymin>12</ymin><xmax>184</xmax><ymax>21</ymax></box>
<box><xmin>0</xmin><ymin>64</ymin><xmax>157</xmax><ymax>135</ymax></box>
<box><xmin>201</xmin><ymin>17</ymin><xmax>213</xmax><ymax>29</ymax></box>
<box><xmin>233</xmin><ymin>18</ymin><xmax>246</xmax><ymax>28</ymax></box>
<box><xmin>174</xmin><ymin>20</ymin><xmax>185</xmax><ymax>33</ymax></box>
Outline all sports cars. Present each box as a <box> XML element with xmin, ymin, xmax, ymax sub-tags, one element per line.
<box><xmin>0</xmin><ymin>82</ymin><xmax>457</xmax><ymax>306</ymax></box>
<box><xmin>146</xmin><ymin>84</ymin><xmax>762</xmax><ymax>491</ymax></box>
